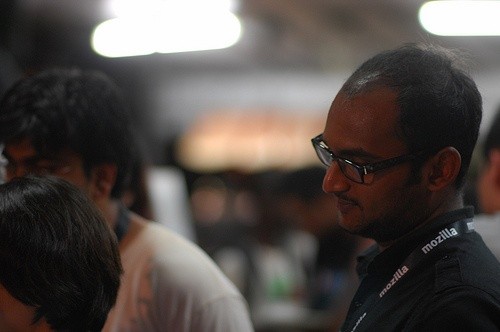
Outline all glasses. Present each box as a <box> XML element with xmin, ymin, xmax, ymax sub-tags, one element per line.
<box><xmin>310</xmin><ymin>134</ymin><xmax>408</xmax><ymax>183</ymax></box>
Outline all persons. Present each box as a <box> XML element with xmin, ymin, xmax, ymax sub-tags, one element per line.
<box><xmin>313</xmin><ymin>42</ymin><xmax>500</xmax><ymax>332</ymax></box>
<box><xmin>0</xmin><ymin>68</ymin><xmax>255</xmax><ymax>332</ymax></box>
<box><xmin>214</xmin><ymin>106</ymin><xmax>500</xmax><ymax>332</ymax></box>
